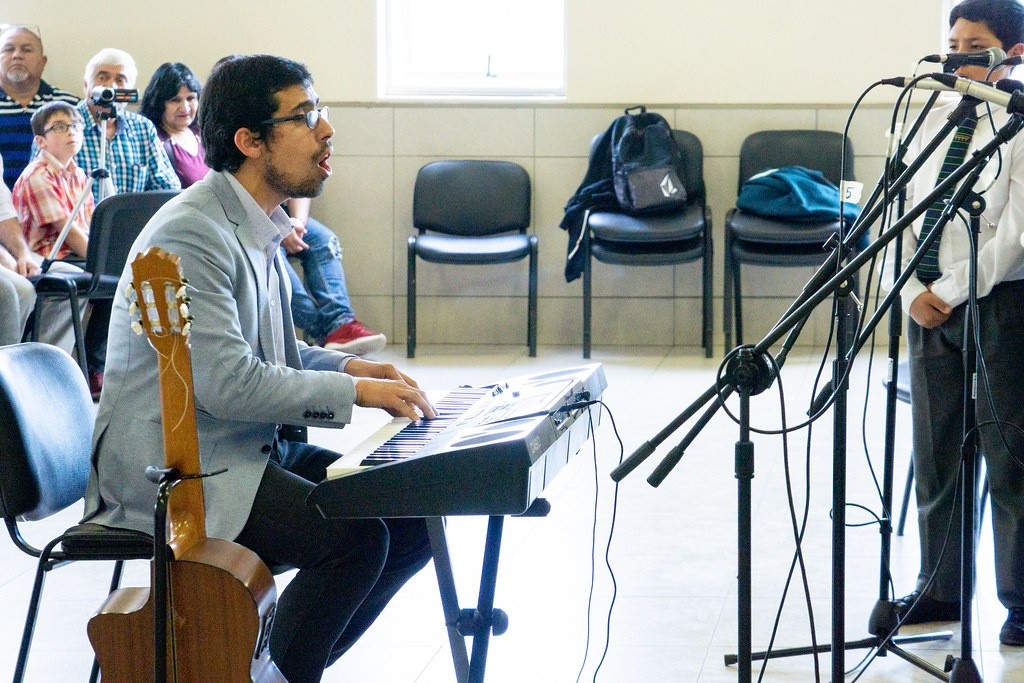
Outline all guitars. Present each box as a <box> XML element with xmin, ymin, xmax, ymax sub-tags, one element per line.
<box><xmin>81</xmin><ymin>245</ymin><xmax>291</xmax><ymax>683</ymax></box>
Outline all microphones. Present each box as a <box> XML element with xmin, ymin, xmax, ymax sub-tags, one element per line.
<box><xmin>882</xmin><ymin>46</ymin><xmax>1024</xmax><ymax>117</ymax></box>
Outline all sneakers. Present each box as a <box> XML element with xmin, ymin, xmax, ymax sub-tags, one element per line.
<box><xmin>323</xmin><ymin>320</ymin><xmax>385</xmax><ymax>355</ymax></box>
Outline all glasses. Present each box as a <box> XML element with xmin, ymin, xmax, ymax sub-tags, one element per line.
<box><xmin>42</xmin><ymin>122</ymin><xmax>82</xmax><ymax>136</ymax></box>
<box><xmin>260</xmin><ymin>106</ymin><xmax>329</xmax><ymax>130</ymax></box>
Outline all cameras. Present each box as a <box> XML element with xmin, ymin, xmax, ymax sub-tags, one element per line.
<box><xmin>92</xmin><ymin>85</ymin><xmax>138</xmax><ymax>102</ymax></box>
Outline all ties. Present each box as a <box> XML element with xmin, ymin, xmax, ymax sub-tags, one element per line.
<box><xmin>916</xmin><ymin>108</ymin><xmax>979</xmax><ymax>285</ymax></box>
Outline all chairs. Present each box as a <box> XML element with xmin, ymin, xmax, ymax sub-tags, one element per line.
<box><xmin>0</xmin><ymin>338</ymin><xmax>297</xmax><ymax>679</ymax></box>
<box><xmin>404</xmin><ymin>160</ymin><xmax>537</xmax><ymax>354</ymax></box>
<box><xmin>576</xmin><ymin>131</ymin><xmax>864</xmax><ymax>357</ymax></box>
<box><xmin>24</xmin><ymin>184</ymin><xmax>192</xmax><ymax>393</ymax></box>
<box><xmin>880</xmin><ymin>359</ymin><xmax>991</xmax><ymax>538</ymax></box>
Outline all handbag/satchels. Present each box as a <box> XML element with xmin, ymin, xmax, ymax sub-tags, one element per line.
<box><xmin>736</xmin><ymin>166</ymin><xmax>869</xmax><ymax>251</ymax></box>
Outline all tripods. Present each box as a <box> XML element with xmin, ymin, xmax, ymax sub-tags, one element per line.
<box><xmin>724</xmin><ymin>63</ymin><xmax>961</xmax><ymax>683</ymax></box>
<box><xmin>41</xmin><ymin>103</ymin><xmax>118</xmax><ymax>279</ymax></box>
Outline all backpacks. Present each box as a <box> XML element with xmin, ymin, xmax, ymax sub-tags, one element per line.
<box><xmin>611</xmin><ymin>107</ymin><xmax>687</xmax><ymax>211</ymax></box>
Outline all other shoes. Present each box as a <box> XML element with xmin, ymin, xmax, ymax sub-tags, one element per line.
<box><xmin>90</xmin><ymin>370</ymin><xmax>106</xmax><ymax>398</ymax></box>
<box><xmin>999</xmin><ymin>608</ymin><xmax>1024</xmax><ymax>644</ymax></box>
<box><xmin>893</xmin><ymin>591</ymin><xmax>962</xmax><ymax>624</ymax></box>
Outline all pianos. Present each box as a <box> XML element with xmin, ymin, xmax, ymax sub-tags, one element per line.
<box><xmin>305</xmin><ymin>362</ymin><xmax>609</xmax><ymax>683</ymax></box>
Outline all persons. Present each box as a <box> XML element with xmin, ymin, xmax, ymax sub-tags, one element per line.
<box><xmin>139</xmin><ymin>61</ymin><xmax>212</xmax><ymax>188</ymax></box>
<box><xmin>79</xmin><ymin>54</ymin><xmax>440</xmax><ymax>683</ymax></box>
<box><xmin>28</xmin><ymin>48</ymin><xmax>182</xmax><ymax>213</ymax></box>
<box><xmin>12</xmin><ymin>101</ymin><xmax>112</xmax><ymax>398</ymax></box>
<box><xmin>210</xmin><ymin>54</ymin><xmax>388</xmax><ymax>357</ymax></box>
<box><xmin>0</xmin><ymin>19</ymin><xmax>81</xmax><ymax>194</ymax></box>
<box><xmin>878</xmin><ymin>1</ymin><xmax>1024</xmax><ymax>647</ymax></box>
<box><xmin>0</xmin><ymin>263</ymin><xmax>37</xmax><ymax>346</ymax></box>
<box><xmin>0</xmin><ymin>152</ymin><xmax>89</xmax><ymax>356</ymax></box>
